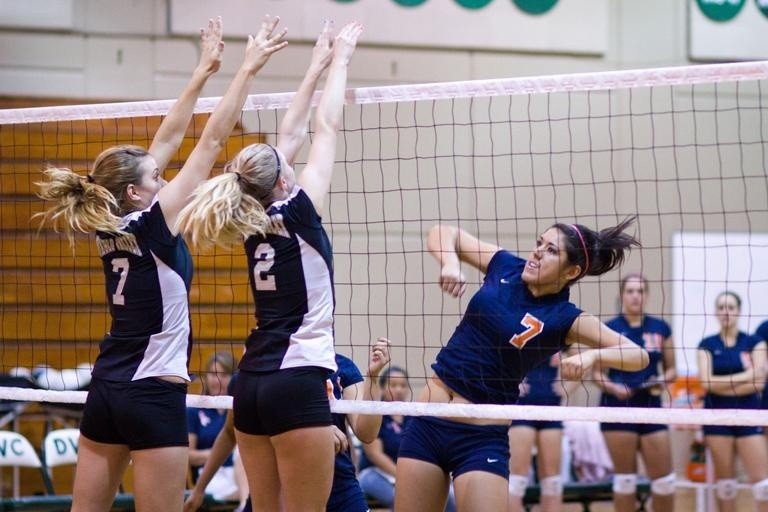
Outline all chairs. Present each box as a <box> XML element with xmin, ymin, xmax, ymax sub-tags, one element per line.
<box><xmin>0</xmin><ymin>427</ymin><xmax>134</xmax><ymax>511</ymax></box>
<box><xmin>524</xmin><ymin>433</ymin><xmax>650</xmax><ymax>511</ymax></box>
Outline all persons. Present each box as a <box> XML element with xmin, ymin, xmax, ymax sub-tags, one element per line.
<box><xmin>185</xmin><ymin>350</ymin><xmax>457</xmax><ymax>512</ymax></box>
<box><xmin>395</xmin><ymin>213</ymin><xmax>768</xmax><ymax>512</ymax></box>
<box><xmin>32</xmin><ymin>14</ymin><xmax>290</xmax><ymax>512</ymax></box>
<box><xmin>174</xmin><ymin>17</ymin><xmax>365</xmax><ymax>511</ymax></box>
